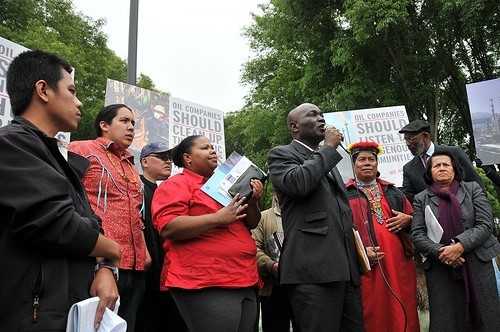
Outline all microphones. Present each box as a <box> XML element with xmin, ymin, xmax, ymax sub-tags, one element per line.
<box><xmin>325</xmin><ymin>125</ymin><xmax>350</xmax><ymax>153</ymax></box>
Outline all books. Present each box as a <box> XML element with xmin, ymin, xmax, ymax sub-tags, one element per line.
<box><xmin>200</xmin><ymin>150</ymin><xmax>268</xmax><ymax>211</ymax></box>
<box><xmin>351</xmin><ymin>226</ymin><xmax>372</xmax><ymax>271</ymax></box>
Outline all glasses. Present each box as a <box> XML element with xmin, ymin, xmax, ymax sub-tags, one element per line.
<box><xmin>147</xmin><ymin>155</ymin><xmax>171</xmax><ymax>161</ymax></box>
<box><xmin>403</xmin><ymin>132</ymin><xmax>428</xmax><ymax>143</ymax></box>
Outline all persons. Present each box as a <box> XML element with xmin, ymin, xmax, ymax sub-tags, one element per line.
<box><xmin>68</xmin><ymin>103</ymin><xmax>152</xmax><ymax>332</ymax></box>
<box><xmin>139</xmin><ymin>141</ymin><xmax>189</xmax><ymax>332</ymax></box>
<box><xmin>0</xmin><ymin>50</ymin><xmax>121</xmax><ymax>332</ymax></box>
<box><xmin>345</xmin><ymin>142</ymin><xmax>421</xmax><ymax>332</ymax></box>
<box><xmin>151</xmin><ymin>135</ymin><xmax>263</xmax><ymax>332</ymax></box>
<box><xmin>251</xmin><ymin>191</ymin><xmax>291</xmax><ymax>332</ymax></box>
<box><xmin>475</xmin><ymin>155</ymin><xmax>500</xmax><ymax>187</ymax></box>
<box><xmin>410</xmin><ymin>151</ymin><xmax>500</xmax><ymax>332</ymax></box>
<box><xmin>267</xmin><ymin>103</ymin><xmax>368</xmax><ymax>332</ymax></box>
<box><xmin>399</xmin><ymin>120</ymin><xmax>488</xmax><ymax>199</ymax></box>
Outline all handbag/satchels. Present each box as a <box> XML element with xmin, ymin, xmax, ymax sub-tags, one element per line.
<box><xmin>228</xmin><ymin>164</ymin><xmax>267</xmax><ymax>205</ymax></box>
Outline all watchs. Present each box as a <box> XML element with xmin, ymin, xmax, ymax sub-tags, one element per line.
<box><xmin>94</xmin><ymin>262</ymin><xmax>120</xmax><ymax>283</ymax></box>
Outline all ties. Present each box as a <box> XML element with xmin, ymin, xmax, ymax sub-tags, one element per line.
<box><xmin>422</xmin><ymin>154</ymin><xmax>429</xmax><ymax>168</ymax></box>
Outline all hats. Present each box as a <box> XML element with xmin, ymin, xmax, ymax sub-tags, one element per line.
<box><xmin>140</xmin><ymin>141</ymin><xmax>172</xmax><ymax>162</ymax></box>
<box><xmin>398</xmin><ymin>120</ymin><xmax>430</xmax><ymax>133</ymax></box>
<box><xmin>348</xmin><ymin>141</ymin><xmax>383</xmax><ymax>156</ymax></box>
<box><xmin>152</xmin><ymin>105</ymin><xmax>166</xmax><ymax>115</ymax></box>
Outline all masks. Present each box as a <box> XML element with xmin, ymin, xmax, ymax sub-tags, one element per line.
<box><xmin>154</xmin><ymin>112</ymin><xmax>160</xmax><ymax>119</ymax></box>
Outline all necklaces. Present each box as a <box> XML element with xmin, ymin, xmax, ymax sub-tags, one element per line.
<box><xmin>105</xmin><ymin>150</ymin><xmax>136</xmax><ymax>184</ymax></box>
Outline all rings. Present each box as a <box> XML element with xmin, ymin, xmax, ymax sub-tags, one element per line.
<box><xmin>396</xmin><ymin>225</ymin><xmax>399</xmax><ymax>229</ymax></box>
<box><xmin>444</xmin><ymin>261</ymin><xmax>449</xmax><ymax>264</ymax></box>
